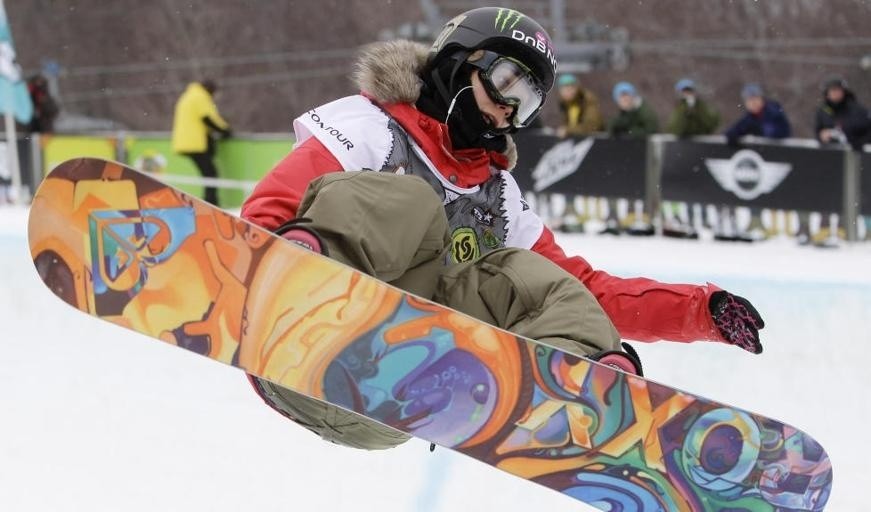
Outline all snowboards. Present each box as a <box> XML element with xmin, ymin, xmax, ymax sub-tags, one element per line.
<box><xmin>29</xmin><ymin>158</ymin><xmax>832</xmax><ymax>512</ymax></box>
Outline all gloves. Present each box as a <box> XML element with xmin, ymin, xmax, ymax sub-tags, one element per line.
<box><xmin>709</xmin><ymin>287</ymin><xmax>769</xmax><ymax>361</ymax></box>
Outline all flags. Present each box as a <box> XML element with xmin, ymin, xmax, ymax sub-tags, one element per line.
<box><xmin>0</xmin><ymin>0</ymin><xmax>35</xmax><ymax>126</ymax></box>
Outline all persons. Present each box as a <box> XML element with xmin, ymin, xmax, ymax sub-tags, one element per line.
<box><xmin>556</xmin><ymin>74</ymin><xmax>605</xmax><ymax>223</ymax></box>
<box><xmin>668</xmin><ymin>78</ymin><xmax>723</xmax><ymax>237</ymax></box>
<box><xmin>725</xmin><ymin>82</ymin><xmax>811</xmax><ymax>246</ymax></box>
<box><xmin>812</xmin><ymin>78</ymin><xmax>871</xmax><ymax>239</ymax></box>
<box><xmin>16</xmin><ymin>76</ymin><xmax>59</xmax><ymax>187</ymax></box>
<box><xmin>608</xmin><ymin>80</ymin><xmax>662</xmax><ymax>228</ymax></box>
<box><xmin>241</xmin><ymin>6</ymin><xmax>764</xmax><ymax>450</ymax></box>
<box><xmin>173</xmin><ymin>79</ymin><xmax>230</xmax><ymax>206</ymax></box>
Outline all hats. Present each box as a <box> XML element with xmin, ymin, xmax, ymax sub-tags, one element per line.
<box><xmin>738</xmin><ymin>81</ymin><xmax>769</xmax><ymax>103</ymax></box>
<box><xmin>817</xmin><ymin>72</ymin><xmax>852</xmax><ymax>98</ymax></box>
<box><xmin>555</xmin><ymin>72</ymin><xmax>579</xmax><ymax>89</ymax></box>
<box><xmin>609</xmin><ymin>81</ymin><xmax>639</xmax><ymax>103</ymax></box>
<box><xmin>673</xmin><ymin>77</ymin><xmax>703</xmax><ymax>98</ymax></box>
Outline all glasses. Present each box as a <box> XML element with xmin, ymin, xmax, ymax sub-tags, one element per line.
<box><xmin>451</xmin><ymin>48</ymin><xmax>545</xmax><ymax>130</ymax></box>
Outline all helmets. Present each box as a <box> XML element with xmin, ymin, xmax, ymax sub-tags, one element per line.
<box><xmin>425</xmin><ymin>6</ymin><xmax>559</xmax><ymax>95</ymax></box>
